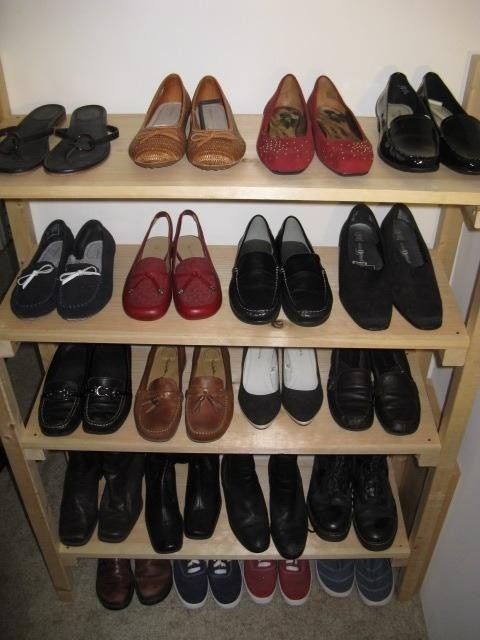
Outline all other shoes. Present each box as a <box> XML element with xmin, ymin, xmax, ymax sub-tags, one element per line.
<box><xmin>39</xmin><ymin>344</ymin><xmax>83</xmax><ymax>436</ymax></box>
<box><xmin>338</xmin><ymin>204</ymin><xmax>393</xmax><ymax>330</ymax></box>
<box><xmin>56</xmin><ymin>219</ymin><xmax>115</xmax><ymax>320</ymax></box>
<box><xmin>307</xmin><ymin>75</ymin><xmax>374</xmax><ymax>175</ymax></box>
<box><xmin>238</xmin><ymin>345</ymin><xmax>281</xmax><ymax>429</ymax></box>
<box><xmin>255</xmin><ymin>74</ymin><xmax>315</xmax><ymax>176</ymax></box>
<box><xmin>11</xmin><ymin>219</ymin><xmax>73</xmax><ymax>320</ymax></box>
<box><xmin>82</xmin><ymin>344</ymin><xmax>132</xmax><ymax>435</ymax></box>
<box><xmin>280</xmin><ymin>347</ymin><xmax>323</xmax><ymax>426</ymax></box>
<box><xmin>186</xmin><ymin>74</ymin><xmax>247</xmax><ymax>171</ymax></box>
<box><xmin>380</xmin><ymin>202</ymin><xmax>442</xmax><ymax>328</ymax></box>
<box><xmin>134</xmin><ymin>345</ymin><xmax>186</xmax><ymax>442</ymax></box>
<box><xmin>184</xmin><ymin>345</ymin><xmax>234</xmax><ymax>443</ymax></box>
<box><xmin>127</xmin><ymin>74</ymin><xmax>192</xmax><ymax>169</ymax></box>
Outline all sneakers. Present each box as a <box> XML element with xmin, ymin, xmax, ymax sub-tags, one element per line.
<box><xmin>97</xmin><ymin>558</ymin><xmax>394</xmax><ymax>610</ymax></box>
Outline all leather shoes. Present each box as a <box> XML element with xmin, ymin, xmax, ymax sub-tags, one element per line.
<box><xmin>171</xmin><ymin>209</ymin><xmax>222</xmax><ymax>321</ymax></box>
<box><xmin>11</xmin><ymin>203</ymin><xmax>443</xmax><ymax>330</ymax></box>
<box><xmin>416</xmin><ymin>72</ymin><xmax>480</xmax><ymax>174</ymax></box>
<box><xmin>228</xmin><ymin>215</ymin><xmax>282</xmax><ymax>325</ymax></box>
<box><xmin>376</xmin><ymin>73</ymin><xmax>441</xmax><ymax>173</ymax></box>
<box><xmin>122</xmin><ymin>211</ymin><xmax>174</xmax><ymax>321</ymax></box>
<box><xmin>275</xmin><ymin>217</ymin><xmax>333</xmax><ymax>328</ymax></box>
<box><xmin>58</xmin><ymin>454</ymin><xmax>398</xmax><ymax>557</ymax></box>
<box><xmin>327</xmin><ymin>348</ymin><xmax>374</xmax><ymax>431</ymax></box>
<box><xmin>369</xmin><ymin>348</ymin><xmax>420</xmax><ymax>436</ymax></box>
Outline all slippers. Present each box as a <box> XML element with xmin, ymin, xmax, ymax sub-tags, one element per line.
<box><xmin>0</xmin><ymin>103</ymin><xmax>67</xmax><ymax>176</ymax></box>
<box><xmin>42</xmin><ymin>105</ymin><xmax>120</xmax><ymax>174</ymax></box>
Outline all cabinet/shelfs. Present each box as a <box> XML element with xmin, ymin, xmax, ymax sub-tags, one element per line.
<box><xmin>1</xmin><ymin>54</ymin><xmax>480</xmax><ymax>606</ymax></box>
